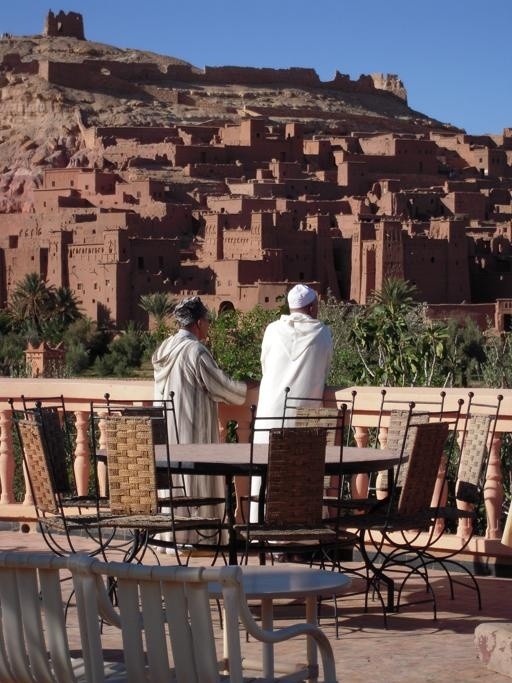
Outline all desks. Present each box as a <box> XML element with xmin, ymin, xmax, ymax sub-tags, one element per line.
<box><xmin>93</xmin><ymin>440</ymin><xmax>412</xmax><ymax>565</ymax></box>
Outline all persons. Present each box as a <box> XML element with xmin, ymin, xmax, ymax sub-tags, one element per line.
<box><xmin>250</xmin><ymin>281</ymin><xmax>333</xmax><ymax>561</ymax></box>
<box><xmin>150</xmin><ymin>298</ymin><xmax>256</xmax><ymax>558</ymax></box>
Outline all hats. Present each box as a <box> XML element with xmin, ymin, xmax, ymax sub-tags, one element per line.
<box><xmin>288</xmin><ymin>284</ymin><xmax>316</xmax><ymax>309</ymax></box>
<box><xmin>173</xmin><ymin>297</ymin><xmax>208</xmax><ymax>327</ymax></box>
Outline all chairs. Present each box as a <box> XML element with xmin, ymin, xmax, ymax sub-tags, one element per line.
<box><xmin>86</xmin><ymin>399</ymin><xmax>223</xmax><ymax>635</ymax></box>
<box><xmin>281</xmin><ymin>387</ymin><xmax>357</xmax><ymax>446</ymax></box>
<box><xmin>322</xmin><ymin>398</ymin><xmax>465</xmax><ymax>630</ymax></box>
<box><xmin>77</xmin><ymin>555</ymin><xmax>341</xmax><ymax>681</ymax></box>
<box><xmin>103</xmin><ymin>389</ymin><xmax>227</xmax><ymax>568</ymax></box>
<box><xmin>2</xmin><ymin>546</ymin><xmax>194</xmax><ymax>680</ymax></box>
<box><xmin>231</xmin><ymin>404</ymin><xmax>345</xmax><ymax>642</ymax></box>
<box><xmin>374</xmin><ymin>390</ymin><xmax>447</xmax><ymax>496</ymax></box>
<box><xmin>8</xmin><ymin>398</ymin><xmax>119</xmax><ymax>628</ymax></box>
<box><xmin>20</xmin><ymin>395</ymin><xmax>122</xmax><ymax>508</ymax></box>
<box><xmin>427</xmin><ymin>391</ymin><xmax>504</xmax><ymax>608</ymax></box>
